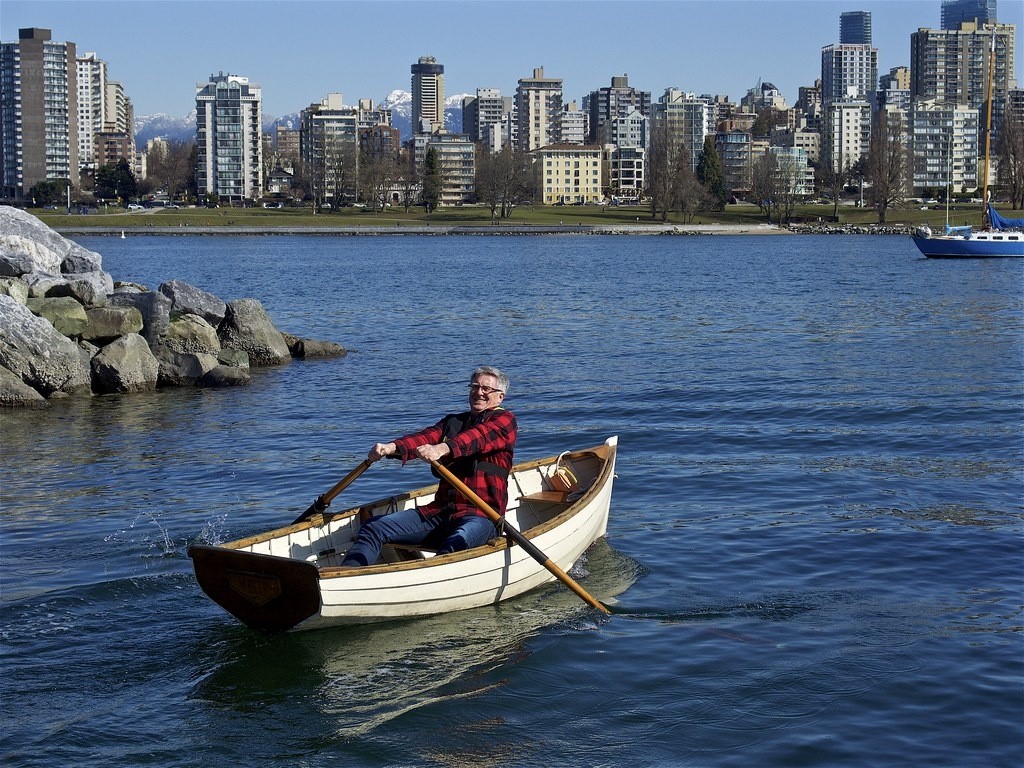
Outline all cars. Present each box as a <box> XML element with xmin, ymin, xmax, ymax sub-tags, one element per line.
<box><xmin>43</xmin><ymin>204</ymin><xmax>57</xmax><ymax>210</ymax></box>
<box><xmin>521</xmin><ymin>200</ymin><xmax>530</xmax><ymax>205</ymax></box>
<box><xmin>551</xmin><ymin>201</ymin><xmax>620</xmax><ymax>207</ymax></box>
<box><xmin>801</xmin><ymin>199</ymin><xmax>831</xmax><ymax>205</ymax></box>
<box><xmin>354</xmin><ymin>203</ymin><xmax>367</xmax><ymax>208</ymax></box>
<box><xmin>380</xmin><ymin>202</ymin><xmax>391</xmax><ymax>207</ymax></box>
<box><xmin>322</xmin><ymin>203</ymin><xmax>336</xmax><ymax>209</ymax></box>
<box><xmin>907</xmin><ymin>196</ymin><xmax>988</xmax><ymax>205</ymax></box>
<box><xmin>77</xmin><ymin>206</ymin><xmax>90</xmax><ymax>215</ymax></box>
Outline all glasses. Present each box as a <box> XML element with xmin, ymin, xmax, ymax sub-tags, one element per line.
<box><xmin>468</xmin><ymin>384</ymin><xmax>503</xmax><ymax>394</ymax></box>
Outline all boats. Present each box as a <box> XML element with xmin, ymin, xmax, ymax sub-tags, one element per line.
<box><xmin>184</xmin><ymin>433</ymin><xmax>619</xmax><ymax>630</ymax></box>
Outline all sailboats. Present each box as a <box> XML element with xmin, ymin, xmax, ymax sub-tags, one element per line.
<box><xmin>910</xmin><ymin>23</ymin><xmax>1024</xmax><ymax>258</ymax></box>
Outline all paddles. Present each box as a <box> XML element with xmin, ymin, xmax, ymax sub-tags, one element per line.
<box><xmin>432</xmin><ymin>460</ymin><xmax>615</xmax><ymax>614</ymax></box>
<box><xmin>289</xmin><ymin>458</ymin><xmax>372</xmax><ymax>525</ymax></box>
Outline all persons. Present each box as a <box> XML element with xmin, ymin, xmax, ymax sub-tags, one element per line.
<box><xmin>338</xmin><ymin>364</ymin><xmax>519</xmax><ymax>573</ymax></box>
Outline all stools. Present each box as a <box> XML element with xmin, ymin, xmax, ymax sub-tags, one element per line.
<box><xmin>387</xmin><ymin>538</ymin><xmax>448</xmax><ymax>557</ymax></box>
<box><xmin>516</xmin><ymin>491</ymin><xmax>581</xmax><ymax>506</ymax></box>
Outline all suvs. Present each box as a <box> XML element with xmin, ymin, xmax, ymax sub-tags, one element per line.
<box><xmin>127</xmin><ymin>204</ymin><xmax>143</xmax><ymax>210</ymax></box>
<box><xmin>164</xmin><ymin>202</ymin><xmax>179</xmax><ymax>209</ymax></box>
